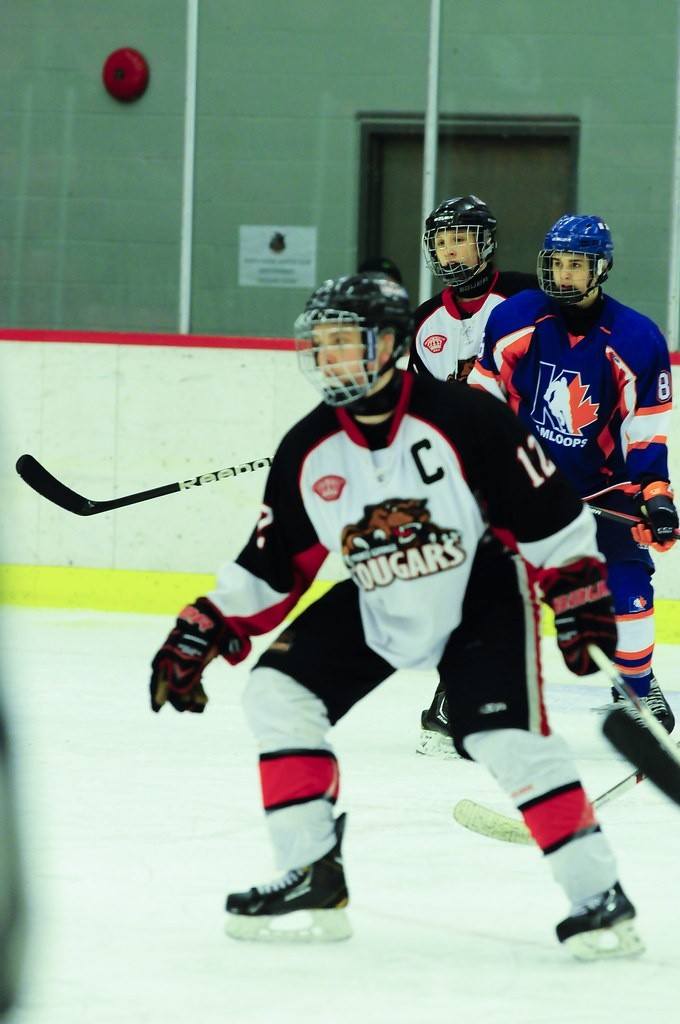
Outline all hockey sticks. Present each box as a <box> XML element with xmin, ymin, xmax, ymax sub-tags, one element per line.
<box><xmin>15</xmin><ymin>452</ymin><xmax>276</xmax><ymax>517</ymax></box>
<box><xmin>452</xmin><ymin>771</ymin><xmax>647</xmax><ymax>845</ymax></box>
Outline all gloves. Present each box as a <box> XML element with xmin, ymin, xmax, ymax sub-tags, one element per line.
<box><xmin>150</xmin><ymin>604</ymin><xmax>223</xmax><ymax>713</ymax></box>
<box><xmin>633</xmin><ymin>480</ymin><xmax>680</xmax><ymax>549</ymax></box>
<box><xmin>547</xmin><ymin>559</ymin><xmax>619</xmax><ymax>673</ymax></box>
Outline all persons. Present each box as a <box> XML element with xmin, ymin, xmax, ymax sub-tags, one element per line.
<box><xmin>396</xmin><ymin>198</ymin><xmax>540</xmax><ymax>751</ymax></box>
<box><xmin>500</xmin><ymin>212</ymin><xmax>675</xmax><ymax>742</ymax></box>
<box><xmin>143</xmin><ymin>274</ymin><xmax>644</xmax><ymax>950</ymax></box>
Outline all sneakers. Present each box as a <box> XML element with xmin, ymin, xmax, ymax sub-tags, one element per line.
<box><xmin>422</xmin><ymin>684</ymin><xmax>469</xmax><ymax>759</ymax></box>
<box><xmin>556</xmin><ymin>881</ymin><xmax>645</xmax><ymax>961</ymax></box>
<box><xmin>610</xmin><ymin>670</ymin><xmax>677</xmax><ymax>740</ymax></box>
<box><xmin>221</xmin><ymin>814</ymin><xmax>353</xmax><ymax>947</ymax></box>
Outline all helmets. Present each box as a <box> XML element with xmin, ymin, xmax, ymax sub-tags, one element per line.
<box><xmin>424</xmin><ymin>193</ymin><xmax>498</xmax><ymax>287</ymax></box>
<box><xmin>536</xmin><ymin>212</ymin><xmax>615</xmax><ymax>303</ymax></box>
<box><xmin>293</xmin><ymin>272</ymin><xmax>416</xmax><ymax>406</ymax></box>
